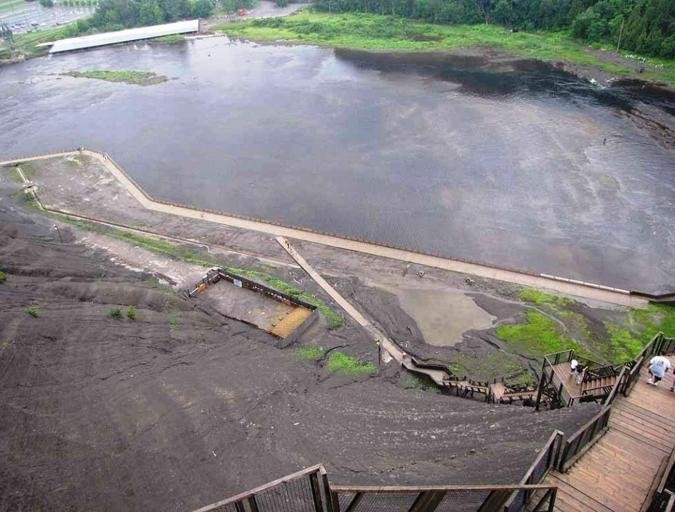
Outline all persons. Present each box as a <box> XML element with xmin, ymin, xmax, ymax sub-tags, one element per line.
<box><xmin>575</xmin><ymin>361</ymin><xmax>586</xmax><ymax>374</ymax></box>
<box><xmin>569</xmin><ymin>358</ymin><xmax>578</xmax><ymax>375</ymax></box>
<box><xmin>670</xmin><ymin>367</ymin><xmax>675</xmax><ymax>392</ymax></box>
<box><xmin>646</xmin><ymin>351</ymin><xmax>671</xmax><ymax>386</ymax></box>
